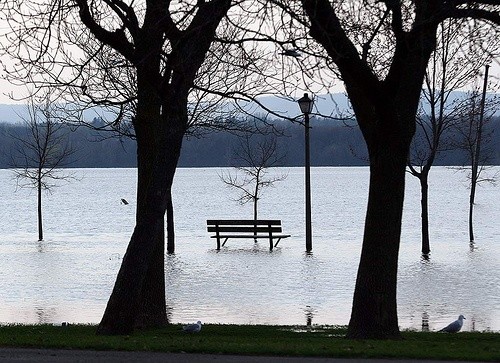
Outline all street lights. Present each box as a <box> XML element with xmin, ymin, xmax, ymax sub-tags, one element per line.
<box><xmin>297</xmin><ymin>94</ymin><xmax>314</xmax><ymax>253</ymax></box>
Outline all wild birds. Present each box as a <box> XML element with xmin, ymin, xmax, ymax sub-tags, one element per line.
<box><xmin>437</xmin><ymin>314</ymin><xmax>467</xmax><ymax>335</ymax></box>
<box><xmin>278</xmin><ymin>49</ymin><xmax>303</xmax><ymax>59</ymax></box>
<box><xmin>182</xmin><ymin>320</ymin><xmax>203</xmax><ymax>334</ymax></box>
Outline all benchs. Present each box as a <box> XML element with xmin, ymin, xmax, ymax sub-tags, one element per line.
<box><xmin>207</xmin><ymin>219</ymin><xmax>290</xmax><ymax>251</ymax></box>
